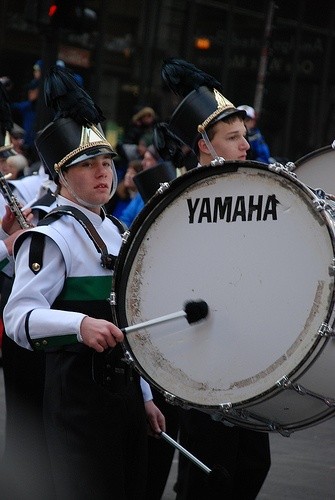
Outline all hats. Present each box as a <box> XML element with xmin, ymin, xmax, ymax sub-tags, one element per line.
<box><xmin>33</xmin><ymin>66</ymin><xmax>118</xmax><ymax>179</ymax></box>
<box><xmin>162</xmin><ymin>58</ymin><xmax>248</xmax><ymax>155</ymax></box>
<box><xmin>0</xmin><ymin>82</ymin><xmax>16</xmax><ymax>158</ymax></box>
<box><xmin>237</xmin><ymin>105</ymin><xmax>255</xmax><ymax>119</ymax></box>
<box><xmin>133</xmin><ymin>162</ymin><xmax>177</xmax><ymax>203</ymax></box>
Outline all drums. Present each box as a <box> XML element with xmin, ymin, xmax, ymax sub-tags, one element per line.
<box><xmin>291</xmin><ymin>140</ymin><xmax>335</xmax><ymax>197</ymax></box>
<box><xmin>108</xmin><ymin>156</ymin><xmax>335</xmax><ymax>438</ymax></box>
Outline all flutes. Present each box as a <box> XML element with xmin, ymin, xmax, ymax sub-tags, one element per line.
<box><xmin>0</xmin><ymin>171</ymin><xmax>30</xmax><ymax>229</ymax></box>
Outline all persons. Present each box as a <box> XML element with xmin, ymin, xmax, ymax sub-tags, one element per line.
<box><xmin>2</xmin><ymin>134</ymin><xmax>166</xmax><ymax>500</ymax></box>
<box><xmin>11</xmin><ymin>78</ymin><xmax>44</xmax><ymax>158</ymax></box>
<box><xmin>0</xmin><ymin>44</ymin><xmax>199</xmax><ymax>500</ymax></box>
<box><xmin>237</xmin><ymin>105</ymin><xmax>270</xmax><ymax>164</ymax></box>
<box><xmin>111</xmin><ymin>160</ymin><xmax>145</xmax><ymax>219</ymax></box>
<box><xmin>173</xmin><ymin>101</ymin><xmax>271</xmax><ymax>500</ymax></box>
<box><xmin>118</xmin><ymin>144</ymin><xmax>166</xmax><ymax>229</ymax></box>
<box><xmin>131</xmin><ymin>104</ymin><xmax>160</xmax><ymax>145</ymax></box>
